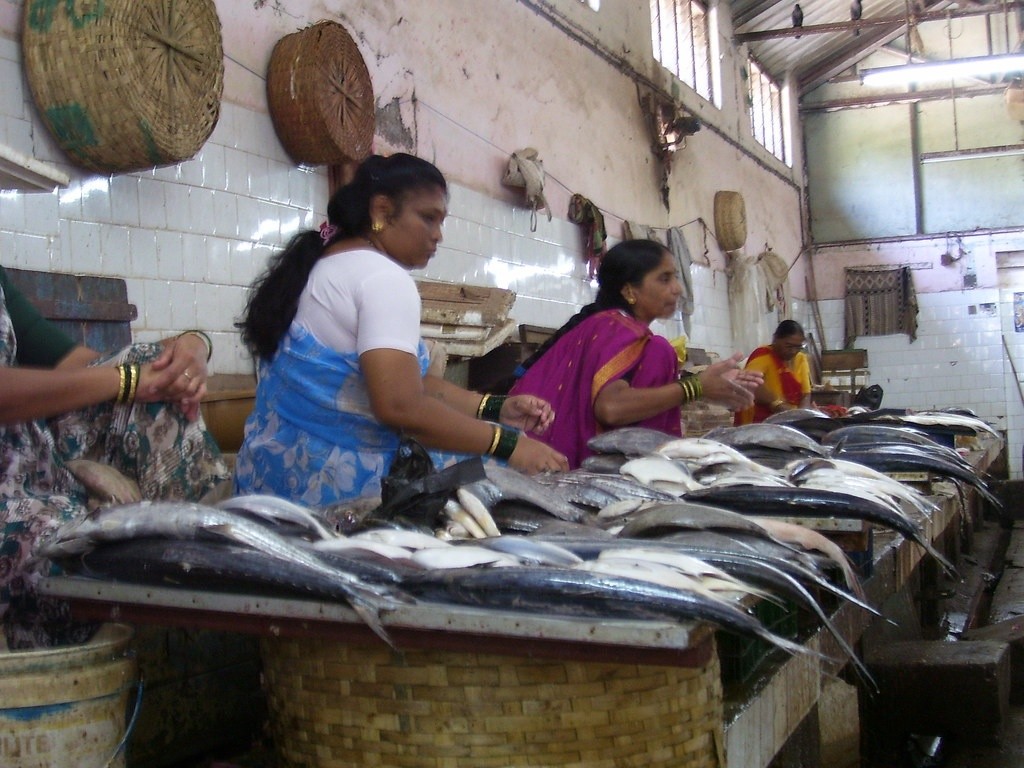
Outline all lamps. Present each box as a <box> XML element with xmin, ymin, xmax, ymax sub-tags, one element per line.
<box><xmin>859</xmin><ymin>49</ymin><xmax>1024</xmax><ymax>85</ymax></box>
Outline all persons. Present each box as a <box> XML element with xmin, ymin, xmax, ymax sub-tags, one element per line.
<box><xmin>235</xmin><ymin>153</ymin><xmax>570</xmax><ymax>516</ymax></box>
<box><xmin>0</xmin><ymin>265</ymin><xmax>228</xmax><ymax>650</ymax></box>
<box><xmin>498</xmin><ymin>239</ymin><xmax>765</xmax><ymax>471</ymax></box>
<box><xmin>733</xmin><ymin>320</ymin><xmax>813</xmax><ymax>427</ymax></box>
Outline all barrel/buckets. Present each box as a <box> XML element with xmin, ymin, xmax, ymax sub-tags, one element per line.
<box><xmin>0</xmin><ymin>621</ymin><xmax>147</xmax><ymax>767</ymax></box>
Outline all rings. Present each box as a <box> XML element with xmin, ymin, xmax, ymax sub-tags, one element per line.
<box><xmin>183</xmin><ymin>369</ymin><xmax>192</xmax><ymax>380</ymax></box>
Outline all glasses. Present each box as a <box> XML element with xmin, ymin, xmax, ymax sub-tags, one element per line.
<box><xmin>781</xmin><ymin>344</ymin><xmax>802</xmax><ymax>352</ymax></box>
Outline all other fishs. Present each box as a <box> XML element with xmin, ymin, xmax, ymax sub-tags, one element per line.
<box><xmin>37</xmin><ymin>411</ymin><xmax>1006</xmax><ymax>693</ymax></box>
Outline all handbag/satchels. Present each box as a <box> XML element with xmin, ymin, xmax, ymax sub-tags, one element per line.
<box><xmin>855</xmin><ymin>384</ymin><xmax>883</xmax><ymax>410</ymax></box>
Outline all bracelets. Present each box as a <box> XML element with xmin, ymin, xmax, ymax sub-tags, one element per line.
<box><xmin>677</xmin><ymin>376</ymin><xmax>703</xmax><ymax>405</ymax></box>
<box><xmin>116</xmin><ymin>364</ymin><xmax>137</xmax><ymax>402</ymax></box>
<box><xmin>177</xmin><ymin>329</ymin><xmax>213</xmax><ymax>363</ymax></box>
<box><xmin>476</xmin><ymin>393</ymin><xmax>508</xmax><ymax>423</ymax></box>
<box><xmin>485</xmin><ymin>422</ymin><xmax>520</xmax><ymax>461</ymax></box>
<box><xmin>771</xmin><ymin>400</ymin><xmax>783</xmax><ymax>407</ymax></box>
